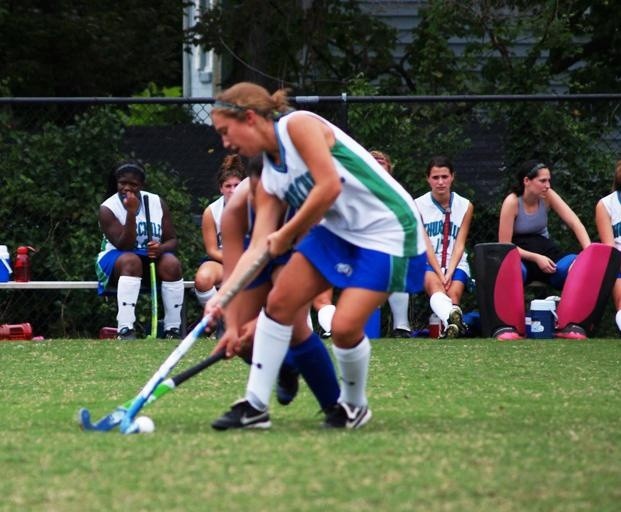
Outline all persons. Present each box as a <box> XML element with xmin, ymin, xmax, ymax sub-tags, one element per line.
<box><xmin>415</xmin><ymin>154</ymin><xmax>474</xmax><ymax>341</ymax></box>
<box><xmin>90</xmin><ymin>158</ymin><xmax>187</xmax><ymax>339</ymax></box>
<box><xmin>594</xmin><ymin>165</ymin><xmax>621</xmax><ymax>335</ymax></box>
<box><xmin>211</xmin><ymin>158</ymin><xmax>352</xmax><ymax>429</ymax></box>
<box><xmin>369</xmin><ymin>150</ymin><xmax>413</xmax><ymax>339</ymax></box>
<box><xmin>212</xmin><ymin>76</ymin><xmax>432</xmax><ymax>431</ymax></box>
<box><xmin>193</xmin><ymin>164</ymin><xmax>338</xmax><ymax>341</ymax></box>
<box><xmin>494</xmin><ymin>158</ymin><xmax>598</xmax><ymax>340</ymax></box>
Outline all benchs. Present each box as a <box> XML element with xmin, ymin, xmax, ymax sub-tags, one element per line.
<box><xmin>406</xmin><ymin>275</ymin><xmax>550</xmax><ymax>338</ymax></box>
<box><xmin>0</xmin><ymin>278</ymin><xmax>225</xmax><ymax>337</ymax></box>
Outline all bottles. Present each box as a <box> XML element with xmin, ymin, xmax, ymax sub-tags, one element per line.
<box><xmin>100</xmin><ymin>327</ymin><xmax>117</xmax><ymax>338</ymax></box>
<box><xmin>16</xmin><ymin>245</ymin><xmax>35</xmax><ymax>282</ymax></box>
<box><xmin>1</xmin><ymin>323</ymin><xmax>32</xmax><ymax>341</ymax></box>
<box><xmin>0</xmin><ymin>245</ymin><xmax>13</xmax><ymax>283</ymax></box>
<box><xmin>430</xmin><ymin>314</ymin><xmax>443</xmax><ymax>338</ymax></box>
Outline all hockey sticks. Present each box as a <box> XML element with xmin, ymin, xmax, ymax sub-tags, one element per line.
<box><xmin>120</xmin><ymin>246</ymin><xmax>272</xmax><ymax>434</ymax></box>
<box><xmin>77</xmin><ymin>340</ymin><xmax>228</xmax><ymax>430</ymax></box>
<box><xmin>141</xmin><ymin>194</ymin><xmax>158</xmax><ymax>339</ymax></box>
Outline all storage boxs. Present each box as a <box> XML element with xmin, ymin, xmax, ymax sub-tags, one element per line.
<box><xmin>0</xmin><ymin>243</ymin><xmax>12</xmax><ymax>283</ymax></box>
<box><xmin>427</xmin><ymin>313</ymin><xmax>444</xmax><ymax>340</ymax></box>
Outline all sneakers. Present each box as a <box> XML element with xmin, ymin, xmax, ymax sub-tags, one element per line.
<box><xmin>446</xmin><ymin>306</ymin><xmax>468</xmax><ymax>335</ymax></box>
<box><xmin>390</xmin><ymin>330</ymin><xmax>413</xmax><ymax>338</ymax></box>
<box><xmin>211</xmin><ymin>360</ymin><xmax>372</xmax><ymax>432</ymax></box>
<box><xmin>115</xmin><ymin>327</ymin><xmax>138</xmax><ymax>340</ymax></box>
<box><xmin>438</xmin><ymin>323</ymin><xmax>457</xmax><ymax>340</ymax></box>
<box><xmin>165</xmin><ymin>328</ymin><xmax>184</xmax><ymax>339</ymax></box>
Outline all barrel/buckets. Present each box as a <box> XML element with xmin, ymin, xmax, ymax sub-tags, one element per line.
<box><xmin>363</xmin><ymin>305</ymin><xmax>382</xmax><ymax>339</ymax></box>
<box><xmin>529</xmin><ymin>299</ymin><xmax>559</xmax><ymax>339</ymax></box>
